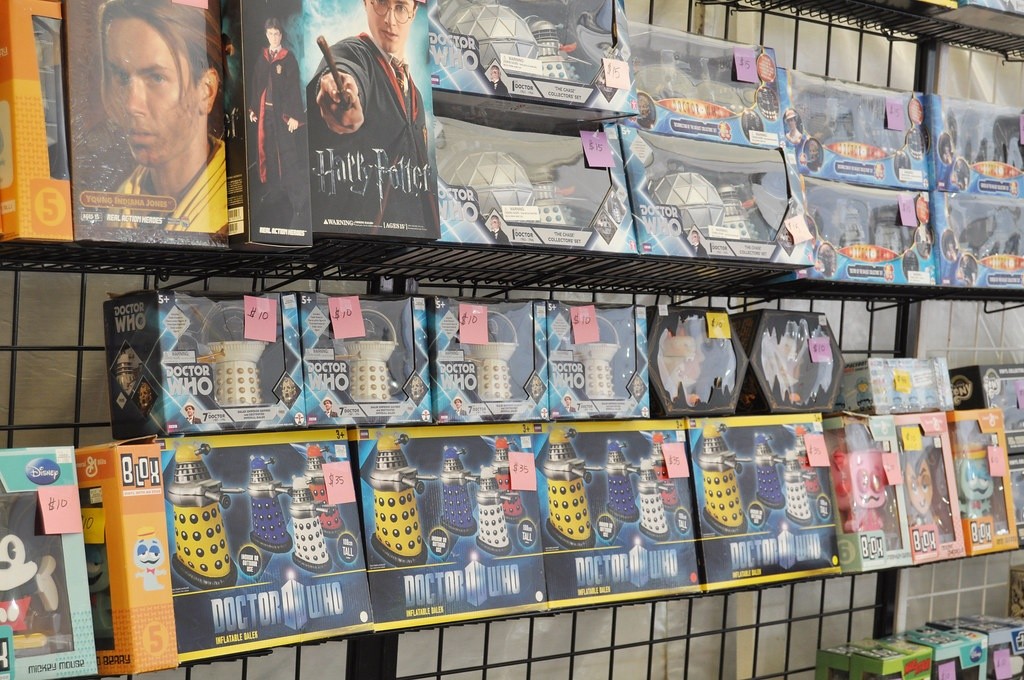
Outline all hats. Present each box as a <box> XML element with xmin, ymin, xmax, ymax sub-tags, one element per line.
<box><xmin>785</xmin><ymin>110</ymin><xmax>796</xmax><ymax>120</ymax></box>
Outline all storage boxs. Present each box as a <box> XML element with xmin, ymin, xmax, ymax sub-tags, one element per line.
<box><xmin>0</xmin><ymin>0</ymin><xmax>1020</xmax><ymax>680</ymax></box>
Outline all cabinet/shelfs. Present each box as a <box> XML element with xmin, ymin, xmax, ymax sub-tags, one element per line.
<box><xmin>1</xmin><ymin>0</ymin><xmax>1024</xmax><ymax>680</ymax></box>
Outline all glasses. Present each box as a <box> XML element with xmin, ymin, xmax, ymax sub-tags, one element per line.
<box><xmin>371</xmin><ymin>0</ymin><xmax>411</xmax><ymax>24</ymax></box>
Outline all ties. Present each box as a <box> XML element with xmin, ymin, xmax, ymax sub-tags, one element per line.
<box><xmin>391</xmin><ymin>61</ymin><xmax>405</xmax><ymax>105</ymax></box>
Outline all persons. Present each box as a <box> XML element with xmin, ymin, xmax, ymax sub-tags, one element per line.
<box><xmin>305</xmin><ymin>0</ymin><xmax>441</xmax><ymax>237</ymax></box>
<box><xmin>564</xmin><ymin>396</ymin><xmax>576</xmax><ymax>412</ymax></box>
<box><xmin>688</xmin><ymin>230</ymin><xmax>708</xmax><ymax>259</ymax></box>
<box><xmin>784</xmin><ymin>109</ymin><xmax>802</xmax><ymax>143</ymax></box>
<box><xmin>807</xmin><ymin>138</ymin><xmax>823</xmax><ymax>171</ymax></box>
<box><xmin>488</xmin><ymin>63</ymin><xmax>508</xmax><ymax>96</ymax></box>
<box><xmin>185</xmin><ymin>405</ymin><xmax>202</xmax><ymax>424</ymax></box>
<box><xmin>454</xmin><ymin>398</ymin><xmax>466</xmax><ymax>416</ymax></box>
<box><xmin>487</xmin><ymin>215</ymin><xmax>509</xmax><ymax>244</ymax></box>
<box><xmin>637</xmin><ymin>90</ymin><xmax>657</xmax><ymax>128</ymax></box>
<box><xmin>248</xmin><ymin>17</ymin><xmax>305</xmax><ymax>243</ymax></box>
<box><xmin>323</xmin><ymin>400</ymin><xmax>338</xmax><ymax>417</ymax></box>
<box><xmin>98</xmin><ymin>0</ymin><xmax>231</xmax><ymax>233</ymax></box>
